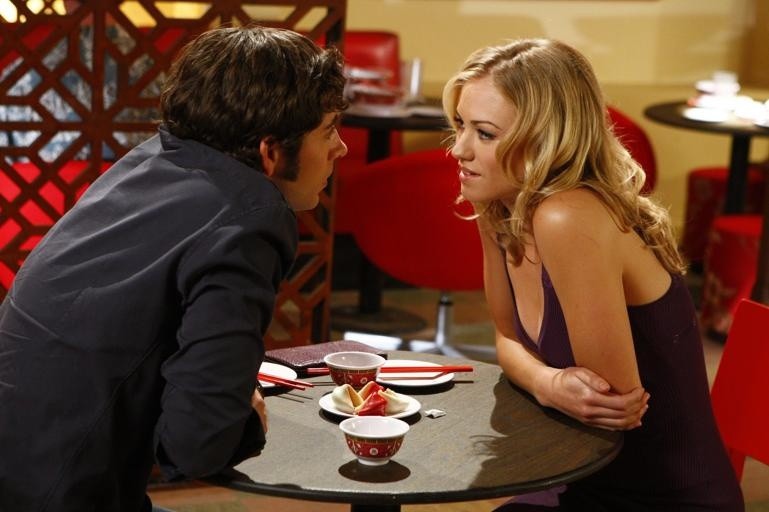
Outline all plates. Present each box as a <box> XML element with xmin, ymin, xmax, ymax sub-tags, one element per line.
<box><xmin>319</xmin><ymin>387</ymin><xmax>421</xmax><ymax>420</ymax></box>
<box><xmin>256</xmin><ymin>361</ymin><xmax>298</xmax><ymax>388</ymax></box>
<box><xmin>372</xmin><ymin>358</ymin><xmax>456</xmax><ymax>389</ymax></box>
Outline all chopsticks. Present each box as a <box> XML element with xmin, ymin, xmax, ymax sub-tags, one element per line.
<box><xmin>256</xmin><ymin>373</ymin><xmax>315</xmax><ymax>391</ymax></box>
<box><xmin>306</xmin><ymin>365</ymin><xmax>474</xmax><ymax>376</ymax></box>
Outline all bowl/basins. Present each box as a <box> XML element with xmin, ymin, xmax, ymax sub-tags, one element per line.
<box><xmin>339</xmin><ymin>415</ymin><xmax>409</xmax><ymax>468</ymax></box>
<box><xmin>323</xmin><ymin>350</ymin><xmax>386</xmax><ymax>390</ymax></box>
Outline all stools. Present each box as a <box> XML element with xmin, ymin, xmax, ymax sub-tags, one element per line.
<box><xmin>684</xmin><ymin>161</ymin><xmax>767</xmax><ymax>340</ymax></box>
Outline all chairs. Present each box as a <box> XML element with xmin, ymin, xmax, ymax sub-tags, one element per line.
<box><xmin>342</xmin><ymin>149</ymin><xmax>499</xmax><ymax>363</ymax></box>
<box><xmin>711</xmin><ymin>298</ymin><xmax>769</xmax><ymax>511</ymax></box>
<box><xmin>607</xmin><ymin>105</ymin><xmax>655</xmax><ymax>197</ymax></box>
<box><xmin>300</xmin><ymin>25</ymin><xmax>403</xmax><ymax>235</ymax></box>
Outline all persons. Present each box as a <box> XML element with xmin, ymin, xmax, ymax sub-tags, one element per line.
<box><xmin>442</xmin><ymin>36</ymin><xmax>747</xmax><ymax>511</ymax></box>
<box><xmin>1</xmin><ymin>25</ymin><xmax>348</xmax><ymax>511</ymax></box>
